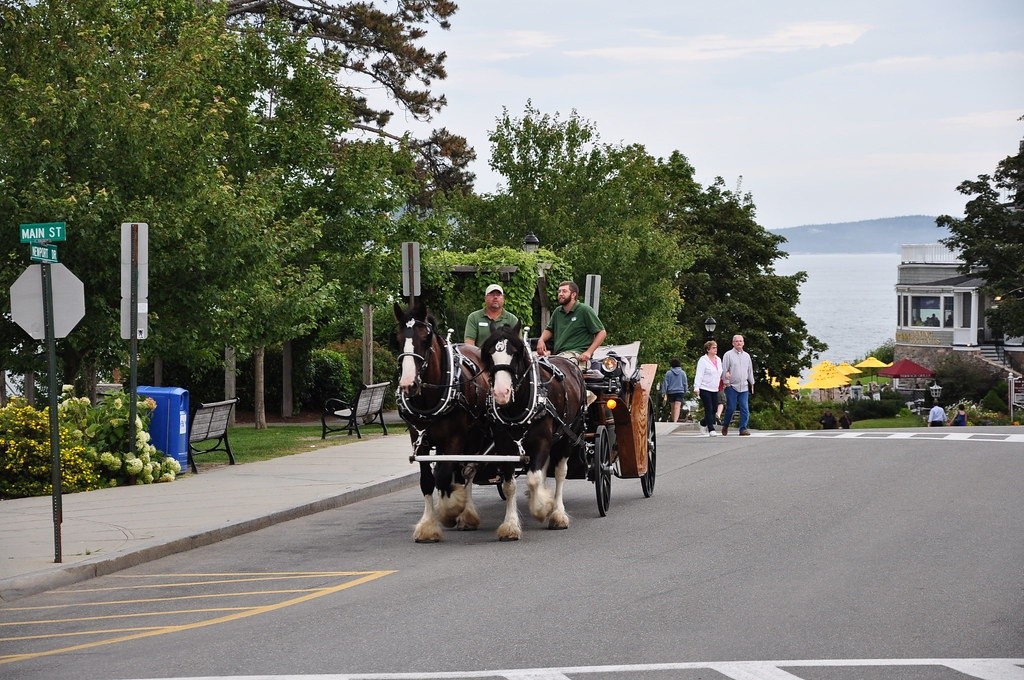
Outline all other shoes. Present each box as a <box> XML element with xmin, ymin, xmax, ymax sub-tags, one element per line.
<box><xmin>710</xmin><ymin>430</ymin><xmax>717</xmax><ymax>437</ymax></box>
<box><xmin>715</xmin><ymin>415</ymin><xmax>722</xmax><ymax>425</ymax></box>
<box><xmin>739</xmin><ymin>430</ymin><xmax>750</xmax><ymax>435</ymax></box>
<box><xmin>698</xmin><ymin>421</ymin><xmax>707</xmax><ymax>435</ymax></box>
<box><xmin>722</xmin><ymin>427</ymin><xmax>728</xmax><ymax>436</ymax></box>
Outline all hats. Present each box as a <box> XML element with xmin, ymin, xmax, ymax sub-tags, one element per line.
<box><xmin>485</xmin><ymin>284</ymin><xmax>503</xmax><ymax>296</ymax></box>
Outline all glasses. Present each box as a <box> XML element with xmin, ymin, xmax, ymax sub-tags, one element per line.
<box><xmin>487</xmin><ymin>294</ymin><xmax>502</xmax><ymax>298</ymax></box>
<box><xmin>709</xmin><ymin>347</ymin><xmax>717</xmax><ymax>349</ymax></box>
<box><xmin>557</xmin><ymin>290</ymin><xmax>570</xmax><ymax>293</ymax></box>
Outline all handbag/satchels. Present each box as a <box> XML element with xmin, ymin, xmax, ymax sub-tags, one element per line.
<box><xmin>950</xmin><ymin>419</ymin><xmax>961</xmax><ymax>426</ymax></box>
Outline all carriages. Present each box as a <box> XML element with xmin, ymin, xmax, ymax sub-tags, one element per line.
<box><xmin>390</xmin><ymin>302</ymin><xmax>660</xmax><ymax>541</ymax></box>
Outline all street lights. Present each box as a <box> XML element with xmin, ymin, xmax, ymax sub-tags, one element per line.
<box><xmin>704</xmin><ymin>316</ymin><xmax>717</xmax><ymax>341</ymax></box>
<box><xmin>929</xmin><ymin>381</ymin><xmax>944</xmax><ymax>402</ymax></box>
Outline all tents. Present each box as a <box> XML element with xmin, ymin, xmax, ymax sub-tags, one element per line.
<box><xmin>877</xmin><ymin>357</ymin><xmax>936</xmax><ymax>393</ymax></box>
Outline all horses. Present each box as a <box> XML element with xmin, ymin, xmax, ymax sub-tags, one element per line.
<box><xmin>392</xmin><ymin>297</ymin><xmax>589</xmax><ymax>543</ymax></box>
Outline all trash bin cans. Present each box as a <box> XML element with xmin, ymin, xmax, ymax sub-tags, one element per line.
<box><xmin>135</xmin><ymin>384</ymin><xmax>189</xmax><ymax>477</ymax></box>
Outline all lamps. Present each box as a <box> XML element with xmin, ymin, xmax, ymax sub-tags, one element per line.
<box><xmin>929</xmin><ymin>381</ymin><xmax>943</xmax><ymax>398</ymax></box>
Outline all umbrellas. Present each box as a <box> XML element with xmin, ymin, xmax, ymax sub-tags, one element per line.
<box><xmin>763</xmin><ymin>356</ymin><xmax>894</xmax><ymax>401</ymax></box>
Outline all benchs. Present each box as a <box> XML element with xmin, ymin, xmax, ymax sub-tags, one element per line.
<box><xmin>187</xmin><ymin>396</ymin><xmax>237</xmax><ymax>472</ymax></box>
<box><xmin>320</xmin><ymin>381</ymin><xmax>390</xmax><ymax>438</ymax></box>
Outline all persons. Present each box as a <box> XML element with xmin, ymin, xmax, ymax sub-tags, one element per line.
<box><xmin>914</xmin><ymin>384</ymin><xmax>922</xmax><ymax>392</ymax></box>
<box><xmin>464</xmin><ymin>284</ymin><xmax>522</xmax><ymax>350</ymax></box>
<box><xmin>661</xmin><ymin>358</ymin><xmax>688</xmax><ymax>423</ymax></box>
<box><xmin>854</xmin><ymin>379</ymin><xmax>863</xmax><ymax>395</ymax></box>
<box><xmin>928</xmin><ymin>398</ymin><xmax>948</xmax><ymax>427</ymax></box>
<box><xmin>839</xmin><ymin>381</ymin><xmax>852</xmax><ymax>397</ymax></box>
<box><xmin>819</xmin><ymin>409</ymin><xmax>852</xmax><ymax>429</ymax></box>
<box><xmin>536</xmin><ymin>280</ymin><xmax>607</xmax><ymax>407</ymax></box>
<box><xmin>912</xmin><ymin>311</ymin><xmax>953</xmax><ymax>327</ymax></box>
<box><xmin>866</xmin><ymin>384</ymin><xmax>878</xmax><ymax>399</ymax></box>
<box><xmin>949</xmin><ymin>404</ymin><xmax>967</xmax><ymax>426</ymax></box>
<box><xmin>693</xmin><ymin>335</ymin><xmax>755</xmax><ymax>437</ymax></box>
